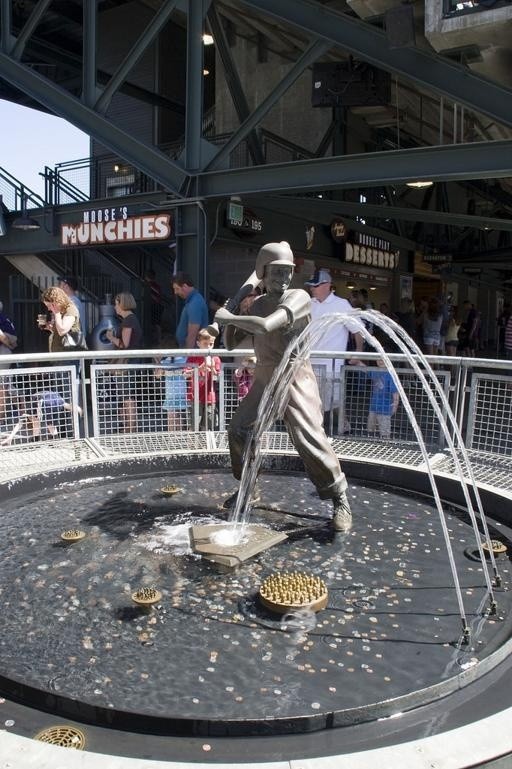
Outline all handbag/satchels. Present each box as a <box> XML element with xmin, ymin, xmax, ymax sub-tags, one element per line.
<box><xmin>106</xmin><ymin>339</ymin><xmax>129</xmax><ymax>374</ymax></box>
<box><xmin>51</xmin><ymin>311</ymin><xmax>87</xmax><ymax>352</ymax></box>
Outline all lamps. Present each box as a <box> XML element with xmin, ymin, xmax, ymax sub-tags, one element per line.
<box><xmin>405</xmin><ymin>95</ymin><xmax>434</xmax><ymax>188</ymax></box>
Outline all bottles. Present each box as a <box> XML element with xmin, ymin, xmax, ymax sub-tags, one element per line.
<box><xmin>107</xmin><ymin>320</ymin><xmax>114</xmax><ymax>336</ymax></box>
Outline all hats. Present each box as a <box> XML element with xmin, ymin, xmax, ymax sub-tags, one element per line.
<box><xmin>303</xmin><ymin>270</ymin><xmax>331</xmax><ymax>287</ymax></box>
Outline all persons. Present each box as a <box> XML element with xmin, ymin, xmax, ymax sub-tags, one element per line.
<box><xmin>0</xmin><ymin>302</ymin><xmax>18</xmax><ymax>421</ymax></box>
<box><xmin>36</xmin><ymin>286</ymin><xmax>84</xmax><ymax>405</ymax></box>
<box><xmin>357</xmin><ymin>345</ymin><xmax>399</xmax><ymax>437</ymax></box>
<box><xmin>105</xmin><ymin>291</ymin><xmax>147</xmax><ymax>431</ymax></box>
<box><xmin>347</xmin><ymin>286</ymin><xmax>511</xmax><ymax>380</ymax></box>
<box><xmin>170</xmin><ymin>271</ymin><xmax>209</xmax><ymax>362</ymax></box>
<box><xmin>208</xmin><ymin>284</ymin><xmax>262</xmax><ymax>350</ymax></box>
<box><xmin>299</xmin><ymin>269</ymin><xmax>368</xmax><ymax>433</ymax></box>
<box><xmin>232</xmin><ymin>355</ymin><xmax>258</xmax><ymax>401</ymax></box>
<box><xmin>57</xmin><ymin>271</ymin><xmax>87</xmax><ymax>340</ymax></box>
<box><xmin>182</xmin><ymin>327</ymin><xmax>222</xmax><ymax>430</ymax></box>
<box><xmin>153</xmin><ymin>332</ymin><xmax>194</xmax><ymax>431</ymax></box>
<box><xmin>195</xmin><ymin>240</ymin><xmax>355</xmax><ymax>533</ymax></box>
<box><xmin>143</xmin><ymin>269</ymin><xmax>165</xmax><ymax>345</ymax></box>
<box><xmin>1</xmin><ymin>390</ymin><xmax>83</xmax><ymax>446</ymax></box>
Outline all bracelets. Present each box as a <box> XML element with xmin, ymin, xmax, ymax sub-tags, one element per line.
<box><xmin>111</xmin><ymin>337</ymin><xmax>114</xmax><ymax>343</ymax></box>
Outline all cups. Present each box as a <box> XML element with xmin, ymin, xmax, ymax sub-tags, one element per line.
<box><xmin>37</xmin><ymin>314</ymin><xmax>47</xmax><ymax>326</ymax></box>
